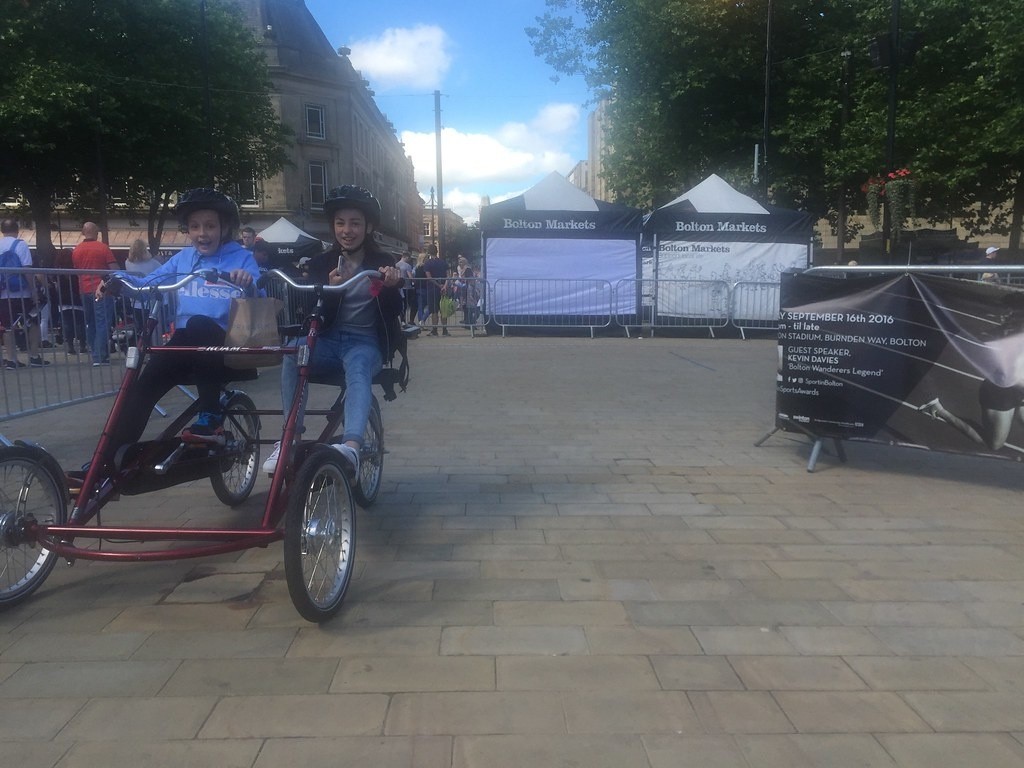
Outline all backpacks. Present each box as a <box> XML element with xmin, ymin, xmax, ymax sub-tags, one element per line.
<box><xmin>0</xmin><ymin>239</ymin><xmax>29</xmax><ymax>293</ymax></box>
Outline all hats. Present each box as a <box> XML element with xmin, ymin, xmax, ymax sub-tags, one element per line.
<box><xmin>172</xmin><ymin>188</ymin><xmax>240</xmax><ymax>230</ymax></box>
<box><xmin>985</xmin><ymin>247</ymin><xmax>999</xmax><ymax>255</ymax></box>
<box><xmin>323</xmin><ymin>184</ymin><xmax>380</xmax><ymax>224</ymax></box>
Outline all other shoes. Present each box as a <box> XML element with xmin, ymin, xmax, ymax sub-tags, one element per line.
<box><xmin>93</xmin><ymin>359</ymin><xmax>112</xmax><ymax>366</ymax></box>
<box><xmin>442</xmin><ymin>330</ymin><xmax>451</xmax><ymax>336</ymax></box>
<box><xmin>418</xmin><ymin>321</ymin><xmax>428</xmax><ymax>330</ymax></box>
<box><xmin>80</xmin><ymin>350</ymin><xmax>91</xmax><ymax>355</ymax></box>
<box><xmin>39</xmin><ymin>340</ymin><xmax>57</xmax><ymax>349</ymax></box>
<box><xmin>67</xmin><ymin>351</ymin><xmax>78</xmax><ymax>356</ymax></box>
<box><xmin>427</xmin><ymin>330</ymin><xmax>438</xmax><ymax>337</ymax></box>
<box><xmin>918</xmin><ymin>398</ymin><xmax>943</xmax><ymax>420</ymax></box>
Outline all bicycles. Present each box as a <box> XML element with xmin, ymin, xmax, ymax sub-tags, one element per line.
<box><xmin>1</xmin><ymin>268</ymin><xmax>388</xmax><ymax>625</ymax></box>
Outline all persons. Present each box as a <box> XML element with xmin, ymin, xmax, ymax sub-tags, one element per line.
<box><xmin>72</xmin><ymin>222</ymin><xmax>121</xmax><ymax>367</ymax></box>
<box><xmin>31</xmin><ymin>252</ymin><xmax>58</xmax><ymax>350</ymax></box>
<box><xmin>262</xmin><ymin>184</ymin><xmax>402</xmax><ymax>488</ymax></box>
<box><xmin>64</xmin><ymin>188</ymin><xmax>268</xmax><ymax>502</ymax></box>
<box><xmin>918</xmin><ymin>283</ymin><xmax>1024</xmax><ymax>452</ymax></box>
<box><xmin>289</xmin><ymin>256</ymin><xmax>321</xmax><ymax>329</ymax></box>
<box><xmin>57</xmin><ymin>248</ymin><xmax>91</xmax><ymax>356</ymax></box>
<box><xmin>979</xmin><ymin>246</ymin><xmax>1001</xmax><ymax>284</ymax></box>
<box><xmin>0</xmin><ymin>217</ymin><xmax>51</xmax><ymax>370</ymax></box>
<box><xmin>250</xmin><ymin>235</ymin><xmax>272</xmax><ymax>275</ymax></box>
<box><xmin>125</xmin><ymin>239</ymin><xmax>163</xmax><ymax>348</ymax></box>
<box><xmin>395</xmin><ymin>244</ymin><xmax>481</xmax><ymax>338</ymax></box>
<box><xmin>240</xmin><ymin>227</ymin><xmax>257</xmax><ymax>252</ymax></box>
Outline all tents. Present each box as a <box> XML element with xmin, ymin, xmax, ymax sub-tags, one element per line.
<box><xmin>253</xmin><ymin>215</ymin><xmax>336</xmax><ymax>288</ymax></box>
<box><xmin>479</xmin><ymin>171</ymin><xmax>644</xmax><ymax>338</ymax></box>
<box><xmin>639</xmin><ymin>173</ymin><xmax>813</xmax><ymax>339</ymax></box>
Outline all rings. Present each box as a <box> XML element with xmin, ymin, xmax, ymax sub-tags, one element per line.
<box><xmin>241</xmin><ymin>277</ymin><xmax>247</xmax><ymax>282</ymax></box>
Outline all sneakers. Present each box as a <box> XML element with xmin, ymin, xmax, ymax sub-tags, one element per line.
<box><xmin>6</xmin><ymin>360</ymin><xmax>26</xmax><ymax>370</ymax></box>
<box><xmin>331</xmin><ymin>443</ymin><xmax>359</xmax><ymax>488</ymax></box>
<box><xmin>182</xmin><ymin>412</ymin><xmax>226</xmax><ymax>447</ymax></box>
<box><xmin>262</xmin><ymin>440</ymin><xmax>296</xmax><ymax>473</ymax></box>
<box><xmin>31</xmin><ymin>355</ymin><xmax>50</xmax><ymax>367</ymax></box>
<box><xmin>64</xmin><ymin>461</ymin><xmax>120</xmax><ymax>502</ymax></box>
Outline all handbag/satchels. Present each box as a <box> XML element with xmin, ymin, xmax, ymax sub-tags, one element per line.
<box><xmin>439</xmin><ymin>294</ymin><xmax>456</xmax><ymax>319</ymax></box>
<box><xmin>224</xmin><ymin>284</ymin><xmax>285</xmax><ymax>369</ymax></box>
